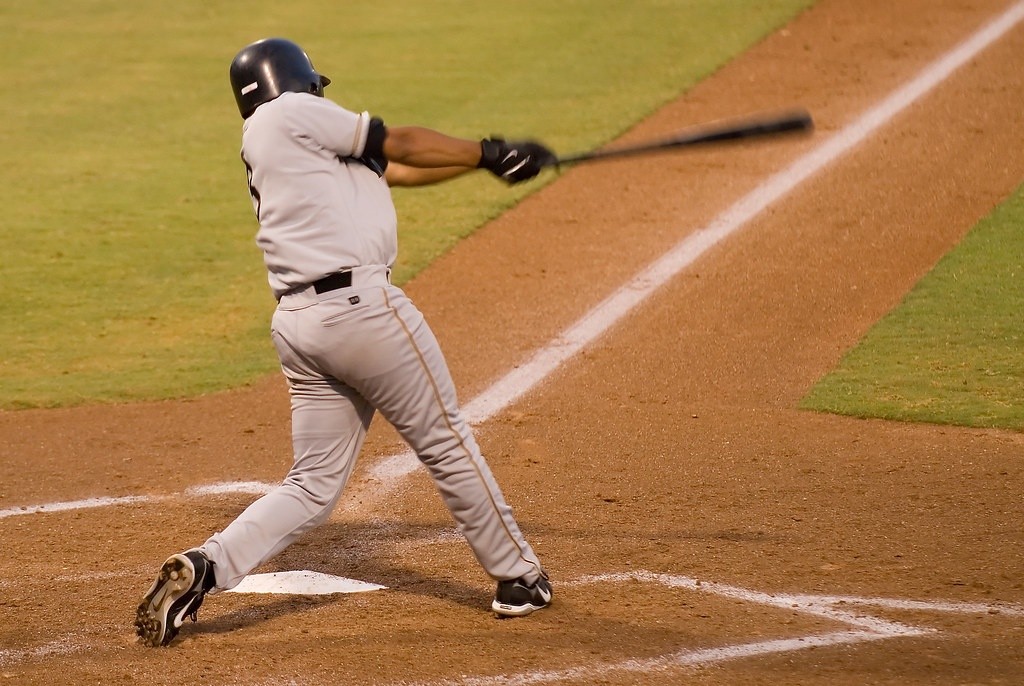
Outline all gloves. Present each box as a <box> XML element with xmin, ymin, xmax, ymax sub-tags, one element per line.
<box><xmin>476</xmin><ymin>138</ymin><xmax>559</xmax><ymax>185</ymax></box>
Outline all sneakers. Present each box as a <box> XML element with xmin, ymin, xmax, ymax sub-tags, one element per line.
<box><xmin>491</xmin><ymin>566</ymin><xmax>552</xmax><ymax>616</ymax></box>
<box><xmin>133</xmin><ymin>548</ymin><xmax>216</xmax><ymax>647</ymax></box>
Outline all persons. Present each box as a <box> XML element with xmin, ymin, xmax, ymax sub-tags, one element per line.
<box><xmin>134</xmin><ymin>36</ymin><xmax>556</xmax><ymax>647</ymax></box>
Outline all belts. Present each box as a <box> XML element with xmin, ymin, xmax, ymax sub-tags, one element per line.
<box><xmin>278</xmin><ymin>270</ymin><xmax>389</xmax><ymax>303</ymax></box>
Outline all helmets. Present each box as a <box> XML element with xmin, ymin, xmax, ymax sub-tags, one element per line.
<box><xmin>230</xmin><ymin>37</ymin><xmax>331</xmax><ymax>119</ymax></box>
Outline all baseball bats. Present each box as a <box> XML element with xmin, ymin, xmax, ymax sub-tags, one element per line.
<box><xmin>538</xmin><ymin>107</ymin><xmax>817</xmax><ymax>172</ymax></box>
